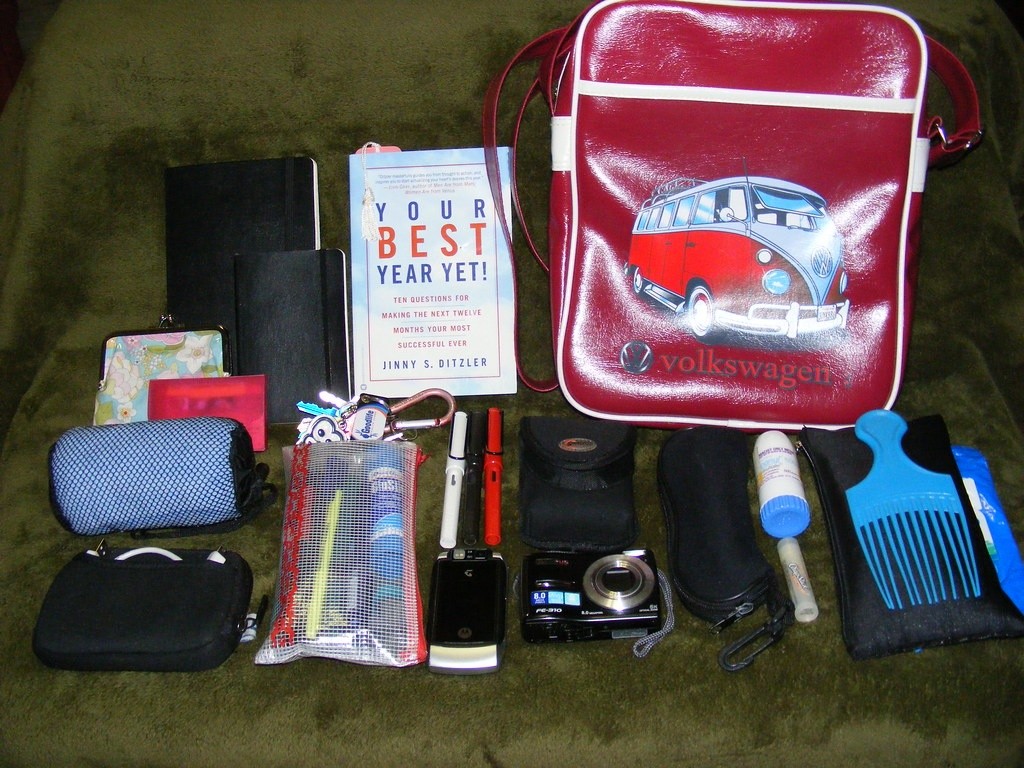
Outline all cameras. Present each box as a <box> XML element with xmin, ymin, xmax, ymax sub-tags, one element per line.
<box><xmin>521</xmin><ymin>548</ymin><xmax>662</xmax><ymax>643</ymax></box>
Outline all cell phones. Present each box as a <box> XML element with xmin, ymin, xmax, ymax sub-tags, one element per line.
<box><xmin>429</xmin><ymin>548</ymin><xmax>507</xmax><ymax>674</ymax></box>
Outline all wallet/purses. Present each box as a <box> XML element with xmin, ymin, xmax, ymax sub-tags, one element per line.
<box><xmin>92</xmin><ymin>324</ymin><xmax>229</xmax><ymax>421</ymax></box>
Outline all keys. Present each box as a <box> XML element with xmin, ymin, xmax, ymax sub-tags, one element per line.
<box><xmin>295</xmin><ymin>390</ymin><xmax>390</xmax><ymax>445</ymax></box>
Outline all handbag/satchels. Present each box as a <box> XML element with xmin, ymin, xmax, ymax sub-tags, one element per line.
<box><xmin>253</xmin><ymin>439</ymin><xmax>427</xmax><ymax>667</ymax></box>
<box><xmin>483</xmin><ymin>0</ymin><xmax>983</xmax><ymax>442</ymax></box>
<box><xmin>49</xmin><ymin>417</ymin><xmax>279</xmax><ymax>537</ymax></box>
<box><xmin>798</xmin><ymin>412</ymin><xmax>1023</xmax><ymax>662</ymax></box>
<box><xmin>33</xmin><ymin>532</ymin><xmax>269</xmax><ymax>671</ymax></box>
<box><xmin>659</xmin><ymin>421</ymin><xmax>794</xmax><ymax>671</ymax></box>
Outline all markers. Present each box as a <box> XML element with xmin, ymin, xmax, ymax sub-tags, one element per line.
<box><xmin>440</xmin><ymin>408</ymin><xmax>504</xmax><ymax>549</ymax></box>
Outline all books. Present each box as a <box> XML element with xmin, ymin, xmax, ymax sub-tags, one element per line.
<box><xmin>161</xmin><ymin>155</ymin><xmax>352</xmax><ymax>425</ymax></box>
